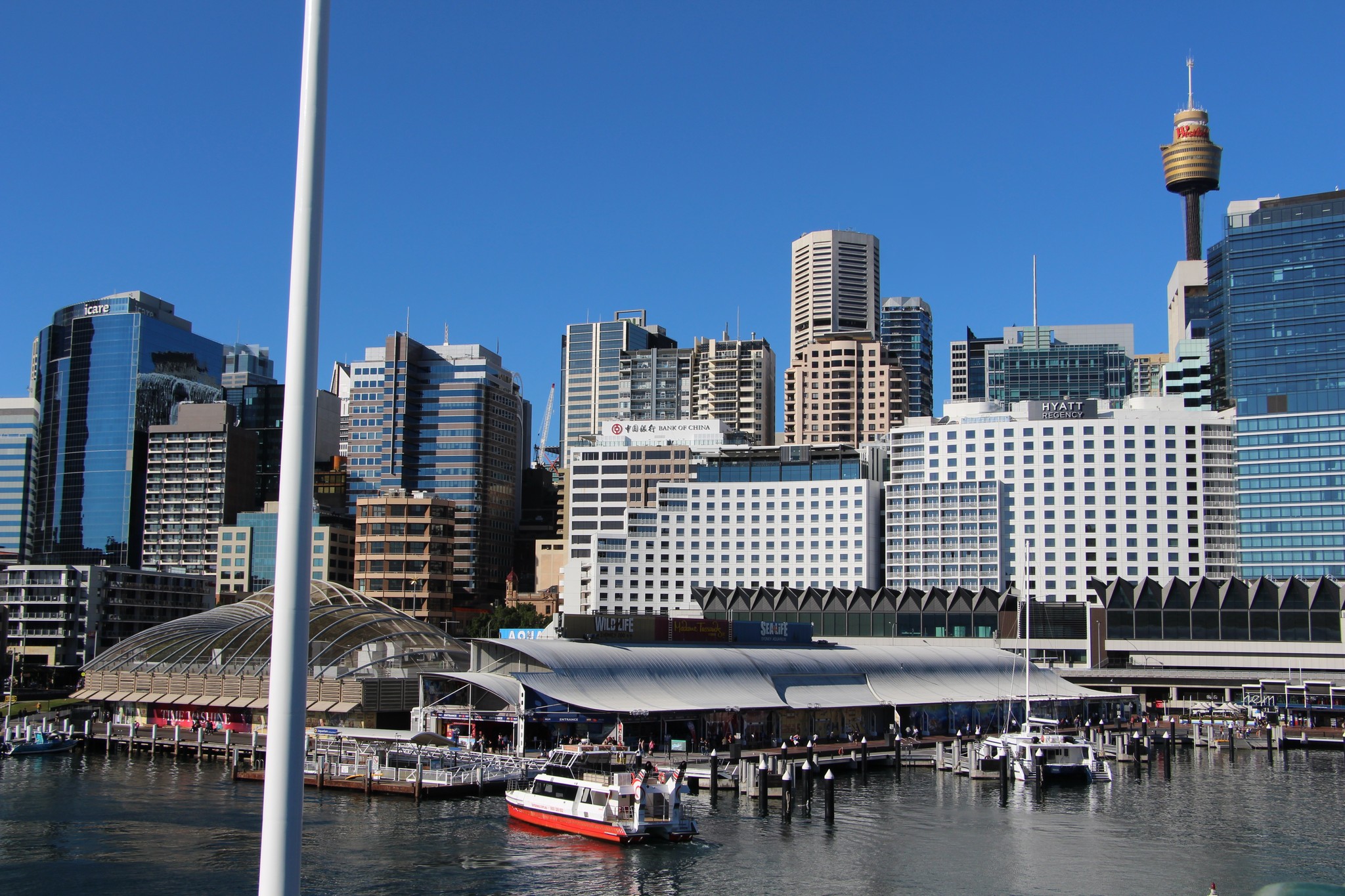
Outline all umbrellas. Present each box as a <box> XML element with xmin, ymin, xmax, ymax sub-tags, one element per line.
<box><xmin>1187</xmin><ymin>701</ymin><xmax>1246</xmax><ymax>717</ymax></box>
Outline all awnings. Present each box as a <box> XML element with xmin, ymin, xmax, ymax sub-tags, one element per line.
<box><xmin>67</xmin><ymin>686</ymin><xmax>359</xmax><ymax>715</ymax></box>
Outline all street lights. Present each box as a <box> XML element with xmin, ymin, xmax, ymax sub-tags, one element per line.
<box><xmin>632</xmin><ymin>709</ymin><xmax>649</xmax><ymax>751</ymax></box>
<box><xmin>1128</xmin><ymin>703</ymin><xmax>1133</xmax><ymax>721</ymax></box>
<box><xmin>394</xmin><ymin>732</ymin><xmax>402</xmax><ymax>781</ymax></box>
<box><xmin>453</xmin><ymin>727</ymin><xmax>460</xmax><ymax>767</ymax></box>
<box><xmin>1274</xmin><ymin>706</ymin><xmax>1279</xmax><ymax>712</ymax></box>
<box><xmin>517</xmin><ymin>710</ymin><xmax>534</xmax><ymax>757</ymax></box>
<box><xmin>1078</xmin><ymin>693</ymin><xmax>1088</xmax><ymax>726</ymax></box>
<box><xmin>431</xmin><ymin>705</ymin><xmax>447</xmax><ymax>747</ymax></box>
<box><xmin>335</xmin><ymin>732</ymin><xmax>342</xmax><ymax>775</ymax></box>
<box><xmin>1047</xmin><ymin>694</ymin><xmax>1058</xmax><ymax>719</ymax></box>
<box><xmin>1096</xmin><ymin>620</ymin><xmax>1101</xmax><ymax>669</ymax></box>
<box><xmin>1304</xmin><ymin>696</ymin><xmax>1316</xmax><ymax>729</ymax></box>
<box><xmin>808</xmin><ymin>703</ymin><xmax>822</xmax><ymax>736</ymax></box>
<box><xmin>941</xmin><ymin>698</ymin><xmax>954</xmax><ymax>736</ymax></box>
<box><xmin>1206</xmin><ymin>695</ymin><xmax>1218</xmax><ymax>726</ymax></box>
<box><xmin>725</xmin><ymin>706</ymin><xmax>740</xmax><ymax>742</ymax></box>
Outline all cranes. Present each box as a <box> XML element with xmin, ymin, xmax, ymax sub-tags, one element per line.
<box><xmin>531</xmin><ymin>382</ymin><xmax>555</xmax><ymax>469</ymax></box>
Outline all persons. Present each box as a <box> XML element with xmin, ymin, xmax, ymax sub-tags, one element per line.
<box><xmin>133</xmin><ymin>721</ymin><xmax>139</xmax><ymax>735</ymax></box>
<box><xmin>894</xmin><ymin>726</ymin><xmax>919</xmax><ymax>740</ymax></box>
<box><xmin>1253</xmin><ymin>715</ymin><xmax>1268</xmax><ymax>729</ymax></box>
<box><xmin>1061</xmin><ymin>715</ymin><xmax>1081</xmax><ymax>731</ymax></box>
<box><xmin>93</xmin><ymin>711</ymin><xmax>111</xmax><ymax>724</ymax></box>
<box><xmin>1342</xmin><ymin>721</ymin><xmax>1345</xmax><ymax>732</ymax></box>
<box><xmin>1299</xmin><ymin>717</ymin><xmax>1306</xmax><ymax>730</ymax></box>
<box><xmin>605</xmin><ymin>737</ymin><xmax>617</xmax><ymax>745</ymax></box>
<box><xmin>18</xmin><ymin>708</ymin><xmax>27</xmax><ymax>722</ymax></box>
<box><xmin>541</xmin><ymin>749</ymin><xmax>553</xmax><ymax>759</ymax></box>
<box><xmin>966</xmin><ymin>724</ymin><xmax>982</xmax><ymax>739</ymax></box>
<box><xmin>638</xmin><ymin>740</ymin><xmax>655</xmax><ymax>758</ymax></box>
<box><xmin>698</xmin><ymin>738</ymin><xmax>709</xmax><ymax>756</ymax></box>
<box><xmin>1220</xmin><ymin>725</ymin><xmax>1250</xmax><ymax>739</ymax></box>
<box><xmin>37</xmin><ymin>702</ymin><xmax>41</xmax><ymax>714</ymax></box>
<box><xmin>1130</xmin><ymin>712</ymin><xmax>1159</xmax><ymax>729</ymax></box>
<box><xmin>558</xmin><ymin>736</ymin><xmax>581</xmax><ymax>748</ymax></box>
<box><xmin>830</xmin><ymin>730</ymin><xmax>859</xmax><ymax>746</ymax></box>
<box><xmin>55</xmin><ymin>711</ymin><xmax>60</xmax><ymax>725</ymax></box>
<box><xmin>790</xmin><ymin>733</ymin><xmax>818</xmax><ymax>747</ymax></box>
<box><xmin>189</xmin><ymin>715</ymin><xmax>213</xmax><ymax>735</ymax></box>
<box><xmin>472</xmin><ymin>733</ymin><xmax>513</xmax><ymax>755</ymax></box>
<box><xmin>722</xmin><ymin>735</ymin><xmax>732</xmax><ymax>751</ymax></box>
<box><xmin>1007</xmin><ymin>718</ymin><xmax>1017</xmax><ymax>733</ymax></box>
<box><xmin>1199</xmin><ymin>723</ymin><xmax>1202</xmax><ymax>735</ymax></box>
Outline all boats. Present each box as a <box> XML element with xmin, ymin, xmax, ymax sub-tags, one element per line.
<box><xmin>0</xmin><ymin>729</ymin><xmax>80</xmax><ymax>755</ymax></box>
<box><xmin>505</xmin><ymin>732</ymin><xmax>700</xmax><ymax>844</ymax></box>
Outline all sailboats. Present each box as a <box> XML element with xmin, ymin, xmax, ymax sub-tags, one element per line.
<box><xmin>971</xmin><ymin>540</ymin><xmax>1114</xmax><ymax>783</ymax></box>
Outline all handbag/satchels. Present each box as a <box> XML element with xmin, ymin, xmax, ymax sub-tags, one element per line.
<box><xmin>502</xmin><ymin>741</ymin><xmax>504</xmax><ymax>744</ymax></box>
<box><xmin>649</xmin><ymin>750</ymin><xmax>652</xmax><ymax>754</ymax></box>
<box><xmin>635</xmin><ymin>750</ymin><xmax>641</xmax><ymax>756</ymax></box>
<box><xmin>705</xmin><ymin>747</ymin><xmax>708</xmax><ymax>750</ymax></box>
<box><xmin>493</xmin><ymin>750</ymin><xmax>496</xmax><ymax>753</ymax></box>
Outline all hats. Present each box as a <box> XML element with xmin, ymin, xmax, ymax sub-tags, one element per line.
<box><xmin>831</xmin><ymin>732</ymin><xmax>834</xmax><ymax>733</ymax></box>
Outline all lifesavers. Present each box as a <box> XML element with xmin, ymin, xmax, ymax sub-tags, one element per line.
<box><xmin>1256</xmin><ymin>731</ymin><xmax>1262</xmax><ymax>736</ymax></box>
<box><xmin>1041</xmin><ymin>736</ymin><xmax>1044</xmax><ymax>742</ymax></box>
<box><xmin>605</xmin><ymin>792</ymin><xmax>612</xmax><ymax>799</ymax></box>
<box><xmin>658</xmin><ymin>773</ymin><xmax>665</xmax><ymax>784</ymax></box>
<box><xmin>631</xmin><ymin>773</ymin><xmax>635</xmax><ymax>783</ymax></box>
<box><xmin>324</xmin><ymin>763</ymin><xmax>328</xmax><ymax>771</ymax></box>
<box><xmin>839</xmin><ymin>748</ymin><xmax>844</xmax><ymax>755</ymax></box>
<box><xmin>985</xmin><ymin>756</ymin><xmax>991</xmax><ymax>759</ymax></box>
